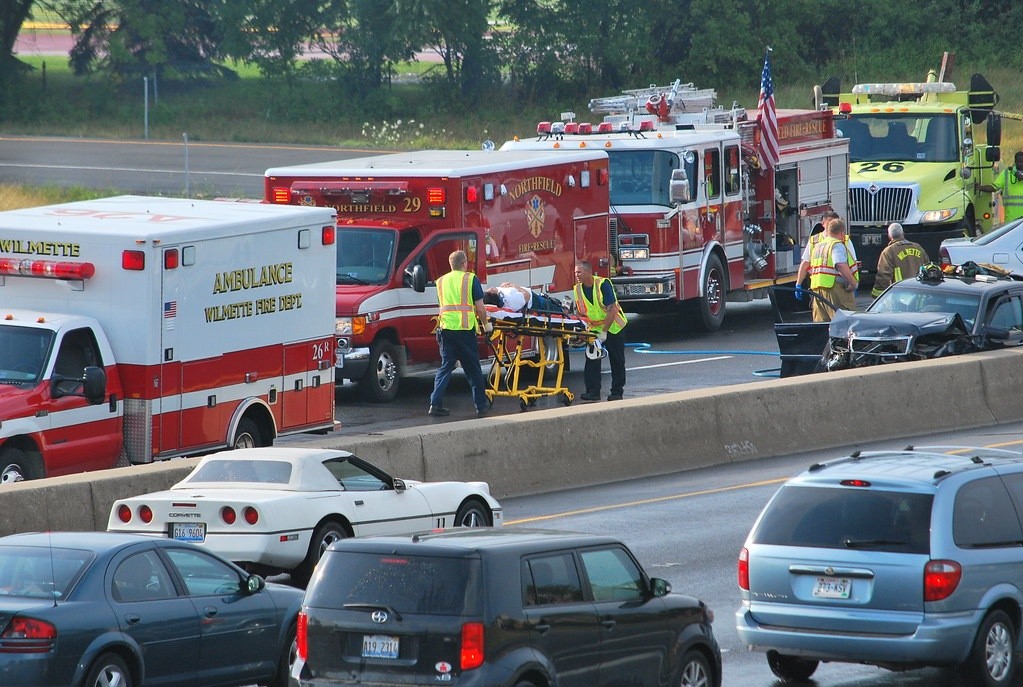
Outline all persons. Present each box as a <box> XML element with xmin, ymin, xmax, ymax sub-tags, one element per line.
<box><xmin>796</xmin><ymin>211</ymin><xmax>860</xmax><ymax>323</ymax></box>
<box><xmin>871</xmin><ymin>223</ymin><xmax>930</xmax><ymax>312</ymax></box>
<box><xmin>573</xmin><ymin>261</ymin><xmax>628</xmax><ymax>401</ymax></box>
<box><xmin>483</xmin><ymin>282</ymin><xmax>572</xmax><ymax>314</ymax></box>
<box><xmin>975</xmin><ymin>151</ymin><xmax>1023</xmax><ymax>225</ymax></box>
<box><xmin>428</xmin><ymin>250</ymin><xmax>494</xmax><ymax>418</ymax></box>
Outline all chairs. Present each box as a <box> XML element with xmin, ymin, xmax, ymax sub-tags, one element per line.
<box><xmin>54</xmin><ymin>345</ymin><xmax>88</xmax><ymax>390</ymax></box>
<box><xmin>926</xmin><ymin>118</ymin><xmax>957</xmax><ymax>160</ymax></box>
<box><xmin>117</xmin><ymin>554</ymin><xmax>154</xmax><ymax>588</ymax></box>
<box><xmin>532</xmin><ymin>561</ymin><xmax>553</xmax><ymax>587</ymax></box>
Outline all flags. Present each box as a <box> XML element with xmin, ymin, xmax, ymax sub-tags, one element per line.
<box><xmin>754</xmin><ymin>46</ymin><xmax>780</xmax><ymax>180</ymax></box>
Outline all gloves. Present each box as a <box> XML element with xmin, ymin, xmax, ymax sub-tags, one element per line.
<box><xmin>795</xmin><ymin>285</ymin><xmax>803</xmax><ymax>301</ymax></box>
<box><xmin>483</xmin><ymin>322</ymin><xmax>493</xmax><ymax>332</ymax></box>
<box><xmin>599</xmin><ymin>331</ymin><xmax>607</xmax><ymax>342</ymax></box>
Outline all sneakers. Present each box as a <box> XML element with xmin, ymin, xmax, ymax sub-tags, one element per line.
<box><xmin>580</xmin><ymin>392</ymin><xmax>601</xmax><ymax>400</ymax></box>
<box><xmin>476</xmin><ymin>408</ymin><xmax>488</xmax><ymax>415</ymax></box>
<box><xmin>428</xmin><ymin>405</ymin><xmax>449</xmax><ymax>416</ymax></box>
<box><xmin>607</xmin><ymin>394</ymin><xmax>623</xmax><ymax>401</ymax></box>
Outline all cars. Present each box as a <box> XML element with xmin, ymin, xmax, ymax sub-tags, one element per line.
<box><xmin>288</xmin><ymin>527</ymin><xmax>722</xmax><ymax>687</ymax></box>
<box><xmin>938</xmin><ymin>213</ymin><xmax>1023</xmax><ymax>276</ymax></box>
<box><xmin>765</xmin><ymin>273</ymin><xmax>1023</xmax><ymax>380</ymax></box>
<box><xmin>0</xmin><ymin>530</ymin><xmax>306</xmax><ymax>687</ymax></box>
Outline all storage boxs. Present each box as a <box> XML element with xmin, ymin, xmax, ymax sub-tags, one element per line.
<box><xmin>777</xmin><ymin>250</ymin><xmax>794</xmax><ymax>268</ymax></box>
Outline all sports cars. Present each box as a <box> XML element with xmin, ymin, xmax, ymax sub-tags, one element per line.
<box><xmin>102</xmin><ymin>447</ymin><xmax>504</xmax><ymax>591</ymax></box>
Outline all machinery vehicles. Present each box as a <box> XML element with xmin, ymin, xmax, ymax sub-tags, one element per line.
<box><xmin>811</xmin><ymin>47</ymin><xmax>1002</xmax><ymax>282</ymax></box>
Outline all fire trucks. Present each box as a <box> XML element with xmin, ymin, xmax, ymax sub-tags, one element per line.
<box><xmin>500</xmin><ymin>79</ymin><xmax>850</xmax><ymax>333</ymax></box>
<box><xmin>260</xmin><ymin>150</ymin><xmax>611</xmax><ymax>406</ymax></box>
<box><xmin>0</xmin><ymin>193</ymin><xmax>343</xmax><ymax>487</ymax></box>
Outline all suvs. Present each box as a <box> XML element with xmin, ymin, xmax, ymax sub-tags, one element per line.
<box><xmin>734</xmin><ymin>445</ymin><xmax>1023</xmax><ymax>687</ymax></box>
